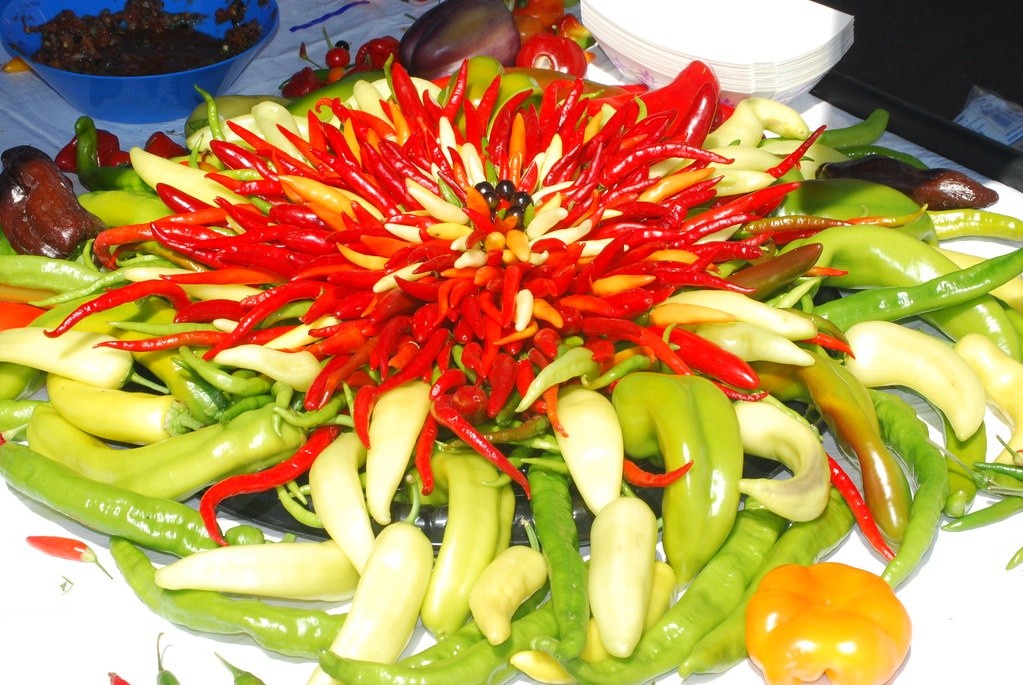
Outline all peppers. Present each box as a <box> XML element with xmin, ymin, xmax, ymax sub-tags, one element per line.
<box><xmin>1</xmin><ymin>0</ymin><xmax>1023</xmax><ymax>685</ymax></box>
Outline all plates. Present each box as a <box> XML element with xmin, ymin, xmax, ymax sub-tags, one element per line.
<box><xmin>578</xmin><ymin>0</ymin><xmax>856</xmax><ymax>121</ymax></box>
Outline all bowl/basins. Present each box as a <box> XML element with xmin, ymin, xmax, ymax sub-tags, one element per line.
<box><xmin>0</xmin><ymin>0</ymin><xmax>280</xmax><ymax>124</ymax></box>
